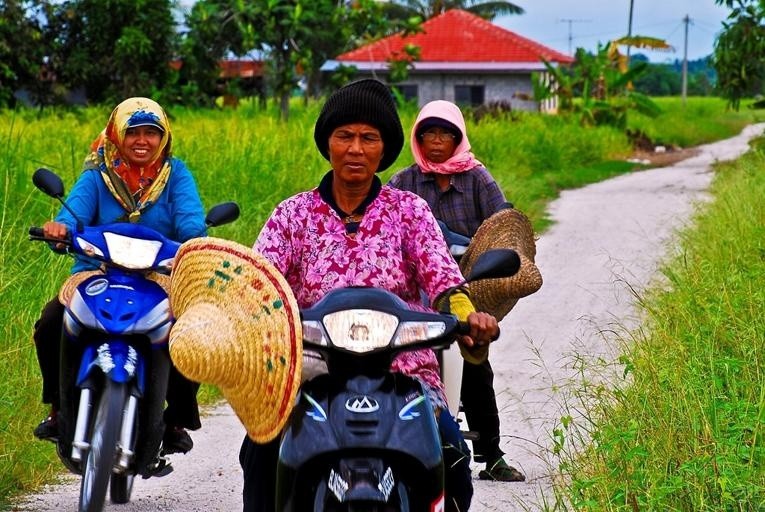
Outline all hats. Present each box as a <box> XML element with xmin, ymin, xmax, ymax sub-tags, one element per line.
<box><xmin>315</xmin><ymin>80</ymin><xmax>404</xmax><ymax>171</ymax></box>
<box><xmin>168</xmin><ymin>237</ymin><xmax>304</xmax><ymax>444</ymax></box>
<box><xmin>125</xmin><ymin>119</ymin><xmax>165</xmax><ymax>132</ymax></box>
<box><xmin>415</xmin><ymin>118</ymin><xmax>462</xmax><ymax>146</ymax></box>
<box><xmin>455</xmin><ymin>209</ymin><xmax>542</xmax><ymax>322</ymax></box>
<box><xmin>59</xmin><ymin>269</ymin><xmax>172</xmax><ymax>307</ymax></box>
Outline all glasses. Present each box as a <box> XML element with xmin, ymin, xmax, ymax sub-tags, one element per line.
<box><xmin>421</xmin><ymin>133</ymin><xmax>458</xmax><ymax>142</ymax></box>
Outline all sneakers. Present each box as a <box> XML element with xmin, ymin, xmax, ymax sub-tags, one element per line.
<box><xmin>163</xmin><ymin>427</ymin><xmax>192</xmax><ymax>452</ymax></box>
<box><xmin>34</xmin><ymin>409</ymin><xmax>56</xmax><ymax>438</ymax></box>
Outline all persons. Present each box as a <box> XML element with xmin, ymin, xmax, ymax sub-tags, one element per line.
<box><xmin>31</xmin><ymin>97</ymin><xmax>209</xmax><ymax>450</ymax></box>
<box><xmin>386</xmin><ymin>101</ymin><xmax>525</xmax><ymax>482</ymax></box>
<box><xmin>238</xmin><ymin>80</ymin><xmax>497</xmax><ymax>511</ymax></box>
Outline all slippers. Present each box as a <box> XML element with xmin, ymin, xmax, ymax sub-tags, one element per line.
<box><xmin>479</xmin><ymin>466</ymin><xmax>525</xmax><ymax>481</ymax></box>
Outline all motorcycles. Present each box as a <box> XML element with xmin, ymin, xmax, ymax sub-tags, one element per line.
<box><xmin>27</xmin><ymin>169</ymin><xmax>239</xmax><ymax>512</ymax></box>
<box><xmin>272</xmin><ymin>220</ymin><xmax>521</xmax><ymax>510</ymax></box>
